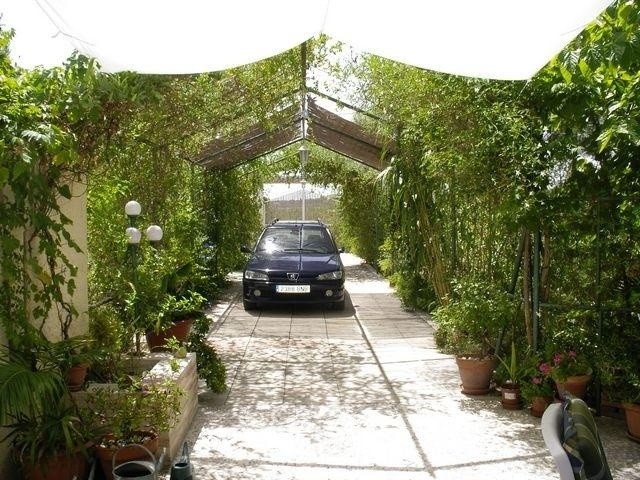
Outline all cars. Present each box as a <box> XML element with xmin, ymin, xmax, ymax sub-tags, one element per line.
<box><xmin>239</xmin><ymin>215</ymin><xmax>346</xmax><ymax>312</ymax></box>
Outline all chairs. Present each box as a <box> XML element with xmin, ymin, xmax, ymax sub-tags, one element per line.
<box><xmin>306</xmin><ymin>234</ymin><xmax>322</xmax><ymax>248</ymax></box>
<box><xmin>541</xmin><ymin>403</ymin><xmax>574</xmax><ymax>480</ymax></box>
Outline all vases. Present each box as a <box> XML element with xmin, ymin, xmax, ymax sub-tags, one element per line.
<box><xmin>554</xmin><ymin>369</ymin><xmax>594</xmax><ymax>400</ymax></box>
<box><xmin>531</xmin><ymin>395</ymin><xmax>553</xmax><ymax>417</ymax></box>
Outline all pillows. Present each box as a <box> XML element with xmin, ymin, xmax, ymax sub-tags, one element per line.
<box><xmin>561</xmin><ymin>398</ymin><xmax>613</xmax><ymax>480</ymax></box>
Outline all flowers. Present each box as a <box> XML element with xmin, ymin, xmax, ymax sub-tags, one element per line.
<box><xmin>551</xmin><ymin>351</ymin><xmax>589</xmax><ymax>384</ymax></box>
<box><xmin>530</xmin><ymin>362</ymin><xmax>554</xmax><ymax>397</ymax></box>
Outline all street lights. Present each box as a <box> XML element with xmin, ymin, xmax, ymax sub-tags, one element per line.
<box><xmin>125</xmin><ymin>197</ymin><xmax>164</xmax><ymax>317</ymax></box>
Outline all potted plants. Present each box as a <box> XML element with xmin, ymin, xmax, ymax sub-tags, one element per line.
<box><xmin>125</xmin><ymin>260</ymin><xmax>207</xmax><ymax>353</ymax></box>
<box><xmin>620</xmin><ymin>374</ymin><xmax>640</xmax><ymax>440</ymax></box>
<box><xmin>83</xmin><ymin>370</ymin><xmax>192</xmax><ymax>480</ymax></box>
<box><xmin>494</xmin><ymin>342</ymin><xmax>533</xmax><ymax>409</ymax></box>
<box><xmin>0</xmin><ymin>351</ymin><xmax>96</xmax><ymax>480</ymax></box>
<box><xmin>52</xmin><ymin>334</ymin><xmax>103</xmax><ymax>391</ymax></box>
<box><xmin>429</xmin><ymin>270</ymin><xmax>517</xmax><ymax>395</ymax></box>
